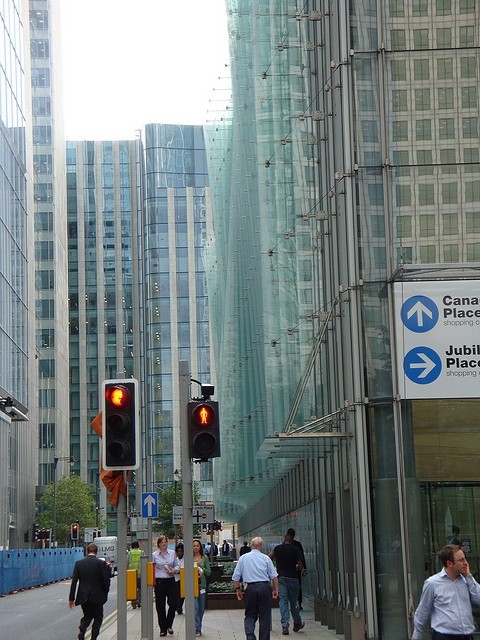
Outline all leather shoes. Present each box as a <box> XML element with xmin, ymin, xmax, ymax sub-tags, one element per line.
<box><xmin>168</xmin><ymin>629</ymin><xmax>173</xmax><ymax>634</ymax></box>
<box><xmin>160</xmin><ymin>633</ymin><xmax>165</xmax><ymax>637</ymax></box>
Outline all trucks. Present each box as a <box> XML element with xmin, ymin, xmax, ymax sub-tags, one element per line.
<box><xmin>93</xmin><ymin>536</ymin><xmax>117</xmax><ymax>567</ymax></box>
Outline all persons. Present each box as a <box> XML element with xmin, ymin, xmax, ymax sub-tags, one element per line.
<box><xmin>240</xmin><ymin>542</ymin><xmax>251</xmax><ymax>556</ymax></box>
<box><xmin>412</xmin><ymin>545</ymin><xmax>480</xmax><ymax>640</ymax></box>
<box><xmin>267</xmin><ymin>533</ymin><xmax>306</xmax><ymax>635</ymax></box>
<box><xmin>205</xmin><ymin>542</ymin><xmax>211</xmax><ymax>554</ymax></box>
<box><xmin>69</xmin><ymin>543</ymin><xmax>111</xmax><ymax>640</ymax></box>
<box><xmin>445</xmin><ymin>525</ymin><xmax>462</xmax><ymax>545</ymax></box>
<box><xmin>213</xmin><ymin>545</ymin><xmax>217</xmax><ymax>556</ymax></box>
<box><xmin>201</xmin><ymin>544</ymin><xmax>209</xmax><ymax>556</ymax></box>
<box><xmin>283</xmin><ymin>527</ymin><xmax>308</xmax><ymax>610</ymax></box>
<box><xmin>151</xmin><ymin>535</ymin><xmax>181</xmax><ymax>636</ymax></box>
<box><xmin>127</xmin><ymin>539</ymin><xmax>146</xmax><ymax>609</ymax></box>
<box><xmin>173</xmin><ymin>543</ymin><xmax>184</xmax><ymax>614</ymax></box>
<box><xmin>222</xmin><ymin>540</ymin><xmax>229</xmax><ymax>556</ymax></box>
<box><xmin>193</xmin><ymin>540</ymin><xmax>212</xmax><ymax>637</ymax></box>
<box><xmin>232</xmin><ymin>536</ymin><xmax>279</xmax><ymax>640</ymax></box>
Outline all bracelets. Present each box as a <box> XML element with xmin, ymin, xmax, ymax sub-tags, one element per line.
<box><xmin>235</xmin><ymin>587</ymin><xmax>240</xmax><ymax>590</ymax></box>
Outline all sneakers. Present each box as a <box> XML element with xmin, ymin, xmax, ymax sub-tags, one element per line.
<box><xmin>293</xmin><ymin>621</ymin><xmax>305</xmax><ymax>632</ymax></box>
<box><xmin>282</xmin><ymin>628</ymin><xmax>289</xmax><ymax>635</ymax></box>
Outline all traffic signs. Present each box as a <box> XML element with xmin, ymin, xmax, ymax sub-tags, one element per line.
<box><xmin>393</xmin><ymin>280</ymin><xmax>480</xmax><ymax>399</ymax></box>
<box><xmin>173</xmin><ymin>506</ymin><xmax>214</xmax><ymax>524</ymax></box>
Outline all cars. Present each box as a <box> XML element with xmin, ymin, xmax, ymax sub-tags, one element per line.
<box><xmin>97</xmin><ymin>557</ymin><xmax>115</xmax><ymax>577</ymax></box>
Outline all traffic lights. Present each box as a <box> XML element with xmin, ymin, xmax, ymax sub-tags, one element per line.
<box><xmin>46</xmin><ymin>530</ymin><xmax>50</xmax><ymax>539</ymax></box>
<box><xmin>42</xmin><ymin>528</ymin><xmax>46</xmax><ymax>539</ymax></box>
<box><xmin>98</xmin><ymin>530</ymin><xmax>102</xmax><ymax>537</ymax></box>
<box><xmin>33</xmin><ymin>523</ymin><xmax>40</xmax><ymax>540</ymax></box>
<box><xmin>24</xmin><ymin>530</ymin><xmax>30</xmax><ymax>542</ymax></box>
<box><xmin>188</xmin><ymin>402</ymin><xmax>220</xmax><ymax>458</ymax></box>
<box><xmin>51</xmin><ymin>528</ymin><xmax>54</xmax><ymax>542</ymax></box>
<box><xmin>101</xmin><ymin>379</ymin><xmax>141</xmax><ymax>471</ymax></box>
<box><xmin>92</xmin><ymin>531</ymin><xmax>97</xmax><ymax>540</ymax></box>
<box><xmin>71</xmin><ymin>523</ymin><xmax>79</xmax><ymax>540</ymax></box>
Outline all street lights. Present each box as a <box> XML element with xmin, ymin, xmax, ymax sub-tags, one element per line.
<box><xmin>96</xmin><ymin>507</ymin><xmax>104</xmax><ymax>527</ymax></box>
<box><xmin>54</xmin><ymin>456</ymin><xmax>75</xmax><ymax>547</ymax></box>
